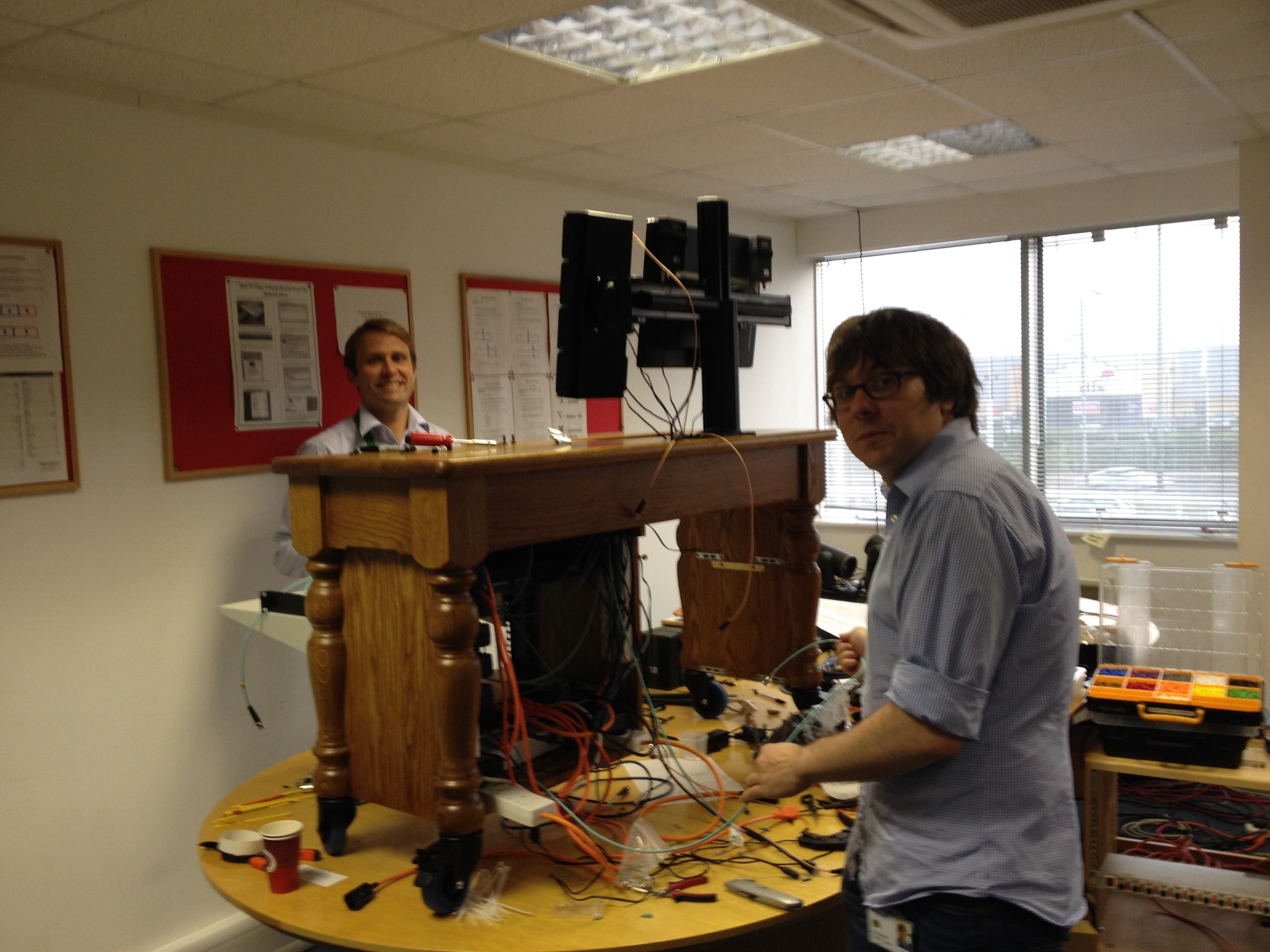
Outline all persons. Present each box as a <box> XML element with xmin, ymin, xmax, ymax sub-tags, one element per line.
<box><xmin>740</xmin><ymin>308</ymin><xmax>1091</xmax><ymax>952</ymax></box>
<box><xmin>272</xmin><ymin>316</ymin><xmax>463</xmax><ymax>579</ymax></box>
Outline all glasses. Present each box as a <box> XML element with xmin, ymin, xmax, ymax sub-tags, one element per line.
<box><xmin>822</xmin><ymin>368</ymin><xmax>921</xmax><ymax>411</ymax></box>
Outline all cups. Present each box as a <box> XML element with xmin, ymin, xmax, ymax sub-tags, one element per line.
<box><xmin>261</xmin><ymin>820</ymin><xmax>304</xmax><ymax>894</ymax></box>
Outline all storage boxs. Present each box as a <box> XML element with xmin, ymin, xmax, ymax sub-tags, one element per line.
<box><xmin>1086</xmin><ymin>552</ymin><xmax>1264</xmax><ymax>757</ymax></box>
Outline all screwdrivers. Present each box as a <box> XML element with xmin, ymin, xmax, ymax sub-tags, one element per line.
<box><xmin>405</xmin><ymin>432</ymin><xmax>497</xmax><ymax>450</ymax></box>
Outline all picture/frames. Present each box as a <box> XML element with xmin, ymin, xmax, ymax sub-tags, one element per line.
<box><xmin>149</xmin><ymin>243</ymin><xmax>421</xmax><ymax>481</ymax></box>
<box><xmin>0</xmin><ymin>235</ymin><xmax>93</xmax><ymax>497</ymax></box>
<box><xmin>459</xmin><ymin>270</ymin><xmax>625</xmax><ymax>451</ymax></box>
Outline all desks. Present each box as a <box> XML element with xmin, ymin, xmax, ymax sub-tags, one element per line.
<box><xmin>272</xmin><ymin>425</ymin><xmax>840</xmax><ymax>918</ymax></box>
<box><xmin>195</xmin><ymin>668</ymin><xmax>856</xmax><ymax>952</ymax></box>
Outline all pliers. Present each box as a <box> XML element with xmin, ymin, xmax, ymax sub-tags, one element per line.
<box><xmin>195</xmin><ymin>842</ymin><xmax>322</xmax><ymax>872</ymax></box>
<box><xmin>650</xmin><ymin>876</ymin><xmax>717</xmax><ymax>902</ymax></box>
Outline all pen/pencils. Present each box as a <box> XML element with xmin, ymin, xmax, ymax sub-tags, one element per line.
<box><xmin>753</xmin><ymin>690</ymin><xmax>786</xmax><ymax>705</ymax></box>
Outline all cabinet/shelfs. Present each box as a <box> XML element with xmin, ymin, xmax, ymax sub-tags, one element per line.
<box><xmin>1083</xmin><ymin>729</ymin><xmax>1270</xmax><ymax>918</ymax></box>
